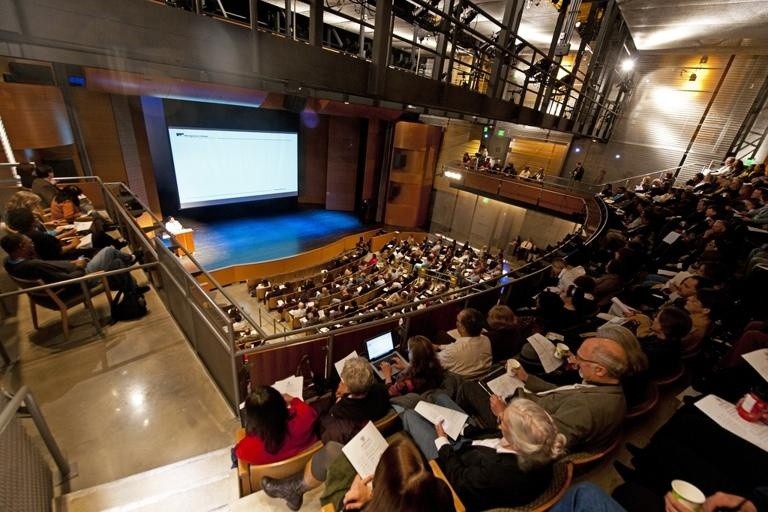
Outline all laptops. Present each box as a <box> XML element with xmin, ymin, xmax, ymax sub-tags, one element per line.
<box><xmin>363</xmin><ymin>330</ymin><xmax>410</xmax><ymax>381</ymax></box>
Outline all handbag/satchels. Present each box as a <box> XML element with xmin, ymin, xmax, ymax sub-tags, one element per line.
<box><xmin>113</xmin><ymin>292</ymin><xmax>147</xmax><ymax>320</ymax></box>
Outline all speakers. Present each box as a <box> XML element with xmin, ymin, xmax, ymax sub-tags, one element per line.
<box><xmin>67</xmin><ymin>75</ymin><xmax>85</xmax><ymax>87</ymax></box>
<box><xmin>400</xmin><ymin>112</ymin><xmax>420</xmax><ymax>122</ymax></box>
<box><xmin>284</xmin><ymin>95</ymin><xmax>307</xmax><ymax>110</ymax></box>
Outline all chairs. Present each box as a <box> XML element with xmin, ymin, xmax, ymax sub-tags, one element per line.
<box><xmin>0</xmin><ymin>185</ymin><xmax>114</xmax><ymax>341</ymax></box>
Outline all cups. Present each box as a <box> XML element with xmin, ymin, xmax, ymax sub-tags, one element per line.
<box><xmin>506</xmin><ymin>358</ymin><xmax>521</xmax><ymax>378</ymax></box>
<box><xmin>669</xmin><ymin>478</ymin><xmax>706</xmax><ymax>512</ymax></box>
<box><xmin>553</xmin><ymin>343</ymin><xmax>569</xmax><ymax>359</ymax></box>
<box><xmin>734</xmin><ymin>392</ymin><xmax>766</xmax><ymax>424</ymax></box>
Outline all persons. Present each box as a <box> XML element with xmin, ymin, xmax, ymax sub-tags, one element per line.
<box><xmin>233</xmin><ymin>143</ymin><xmax>768</xmax><ymax>512</ymax></box>
<box><xmin>0</xmin><ymin>161</ymin><xmax>151</xmax><ymax>312</ymax></box>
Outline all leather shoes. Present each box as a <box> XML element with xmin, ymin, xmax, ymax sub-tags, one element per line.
<box><xmin>262</xmin><ymin>477</ymin><xmax>302</xmax><ymax>511</ymax></box>
<box><xmin>135</xmin><ymin>286</ymin><xmax>149</xmax><ymax>294</ymax></box>
<box><xmin>130</xmin><ymin>251</ymin><xmax>143</xmax><ymax>266</ymax></box>
